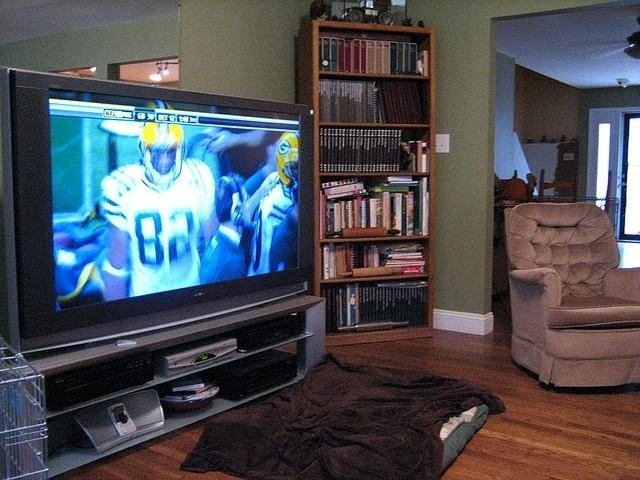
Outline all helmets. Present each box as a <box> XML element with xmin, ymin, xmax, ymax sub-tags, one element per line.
<box><xmin>275</xmin><ymin>131</ymin><xmax>299</xmax><ymax>189</ymax></box>
<box><xmin>138</xmin><ymin>122</ymin><xmax>185</xmax><ymax>184</ymax></box>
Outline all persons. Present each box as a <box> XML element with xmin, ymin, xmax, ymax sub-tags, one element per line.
<box><xmin>250</xmin><ymin>132</ymin><xmax>298</xmax><ymax>273</ymax></box>
<box><xmin>99</xmin><ymin>121</ymin><xmax>216</xmax><ymax>303</ymax></box>
<box><xmin>200</xmin><ymin>172</ymin><xmax>279</xmax><ymax>284</ymax></box>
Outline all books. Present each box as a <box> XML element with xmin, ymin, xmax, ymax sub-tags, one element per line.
<box><xmin>322</xmin><ymin>240</ymin><xmax>426</xmax><ymax>279</ymax></box>
<box><xmin>321</xmin><ymin>280</ymin><xmax>429</xmax><ymax>333</ymax></box>
<box><xmin>319</xmin><ymin>78</ymin><xmax>430</xmax><ymax>125</ymax></box>
<box><xmin>319</xmin><ymin>126</ymin><xmax>430</xmax><ymax>172</ymax></box>
<box><xmin>318</xmin><ymin>34</ymin><xmax>430</xmax><ymax>77</ymax></box>
<box><xmin>319</xmin><ymin>177</ymin><xmax>429</xmax><ymax>238</ymax></box>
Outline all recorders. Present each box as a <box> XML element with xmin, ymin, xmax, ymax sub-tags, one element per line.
<box><xmin>347</xmin><ymin>6</ymin><xmax>393</xmax><ymax>25</ymax></box>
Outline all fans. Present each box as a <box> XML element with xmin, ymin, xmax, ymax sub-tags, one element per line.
<box><xmin>579</xmin><ymin>16</ymin><xmax>640</xmax><ymax>60</ymax></box>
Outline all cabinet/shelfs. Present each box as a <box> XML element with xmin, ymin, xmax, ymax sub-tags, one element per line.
<box><xmin>297</xmin><ymin>19</ymin><xmax>436</xmax><ymax>348</ymax></box>
<box><xmin>0</xmin><ymin>295</ymin><xmax>329</xmax><ymax>480</ymax></box>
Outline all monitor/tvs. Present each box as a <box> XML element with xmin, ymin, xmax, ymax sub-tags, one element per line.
<box><xmin>1</xmin><ymin>65</ymin><xmax>314</xmax><ymax>356</ymax></box>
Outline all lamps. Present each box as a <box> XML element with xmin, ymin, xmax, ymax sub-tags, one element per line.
<box><xmin>148</xmin><ymin>60</ymin><xmax>178</xmax><ymax>83</ymax></box>
<box><xmin>616</xmin><ymin>78</ymin><xmax>629</xmax><ymax>88</ymax></box>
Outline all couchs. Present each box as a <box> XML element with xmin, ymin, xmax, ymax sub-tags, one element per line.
<box><xmin>502</xmin><ymin>202</ymin><xmax>640</xmax><ymax>392</ymax></box>
